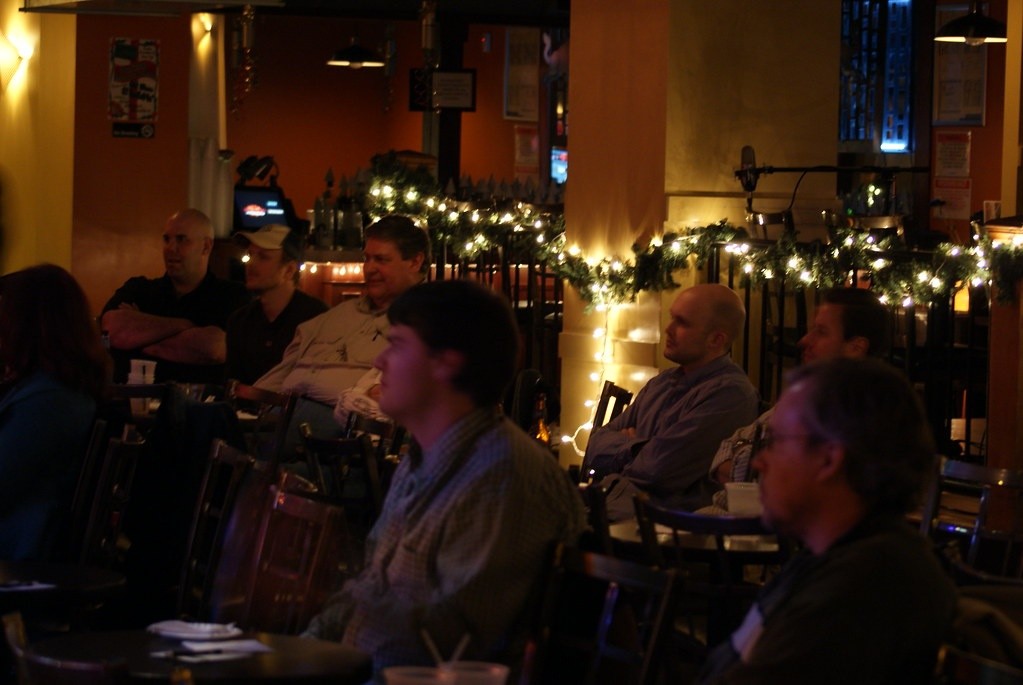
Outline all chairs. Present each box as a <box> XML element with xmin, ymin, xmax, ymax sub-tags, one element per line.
<box><xmin>0</xmin><ymin>368</ymin><xmax>1023</xmax><ymax>685</ymax></box>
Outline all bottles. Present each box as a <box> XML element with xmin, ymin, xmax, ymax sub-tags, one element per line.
<box><xmin>299</xmin><ymin>412</ymin><xmax>360</xmax><ymax>497</ymax></box>
<box><xmin>525</xmin><ymin>391</ymin><xmax>552</xmax><ymax>452</ymax></box>
<box><xmin>93</xmin><ymin>315</ymin><xmax>112</xmax><ymax>355</ymax></box>
<box><xmin>567</xmin><ymin>465</ymin><xmax>607</xmax><ymax>505</ymax></box>
<box><xmin>441</xmin><ymin>171</ymin><xmax>557</xmax><ymax>206</ymax></box>
<box><xmin>304</xmin><ymin>169</ymin><xmax>363</xmax><ymax>252</ymax></box>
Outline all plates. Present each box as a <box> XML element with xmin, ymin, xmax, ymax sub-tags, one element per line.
<box><xmin>148</xmin><ymin>622</ymin><xmax>244</xmax><ymax>640</ymax></box>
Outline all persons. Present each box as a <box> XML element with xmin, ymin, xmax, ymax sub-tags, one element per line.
<box><xmin>299</xmin><ymin>280</ymin><xmax>582</xmax><ymax>685</ymax></box>
<box><xmin>688</xmin><ymin>357</ymin><xmax>943</xmax><ymax>685</ymax></box>
<box><xmin>0</xmin><ymin>208</ymin><xmax>434</xmax><ymax>630</ymax></box>
<box><xmin>573</xmin><ymin>282</ymin><xmax>887</xmax><ymax>537</ymax></box>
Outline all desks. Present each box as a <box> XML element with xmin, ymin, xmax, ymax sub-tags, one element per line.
<box><xmin>22</xmin><ymin>628</ymin><xmax>373</xmax><ymax>685</ymax></box>
<box><xmin>608</xmin><ymin>512</ymin><xmax>779</xmax><ymax>654</ymax></box>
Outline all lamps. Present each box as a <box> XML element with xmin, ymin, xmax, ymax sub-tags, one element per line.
<box><xmin>933</xmin><ymin>0</ymin><xmax>1007</xmax><ymax>47</ymax></box>
<box><xmin>325</xmin><ymin>19</ymin><xmax>386</xmax><ymax>70</ymax></box>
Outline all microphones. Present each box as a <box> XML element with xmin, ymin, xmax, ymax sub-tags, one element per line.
<box><xmin>739</xmin><ymin>146</ymin><xmax>758</xmax><ymax>201</ymax></box>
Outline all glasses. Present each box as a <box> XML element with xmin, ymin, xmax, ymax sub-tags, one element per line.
<box><xmin>764</xmin><ymin>425</ymin><xmax>827</xmax><ymax>450</ymax></box>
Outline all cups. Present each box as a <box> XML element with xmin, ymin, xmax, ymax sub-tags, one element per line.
<box><xmin>383</xmin><ymin>666</ymin><xmax>459</xmax><ymax>685</ymax></box>
<box><xmin>183</xmin><ymin>382</ymin><xmax>204</xmax><ymax>402</ymax></box>
<box><xmin>127</xmin><ymin>358</ymin><xmax>157</xmax><ymax>416</ymax></box>
<box><xmin>723</xmin><ymin>482</ymin><xmax>765</xmax><ymax>541</ymax></box>
<box><xmin>439</xmin><ymin>661</ymin><xmax>510</xmax><ymax>685</ymax></box>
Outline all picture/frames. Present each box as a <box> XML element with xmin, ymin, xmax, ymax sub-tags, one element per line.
<box><xmin>931</xmin><ymin>3</ymin><xmax>990</xmax><ymax>128</ymax></box>
<box><xmin>503</xmin><ymin>27</ymin><xmax>540</xmax><ymax>123</ymax></box>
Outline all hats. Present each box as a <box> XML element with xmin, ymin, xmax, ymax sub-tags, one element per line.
<box><xmin>232</xmin><ymin>223</ymin><xmax>291</xmax><ymax>250</ymax></box>
